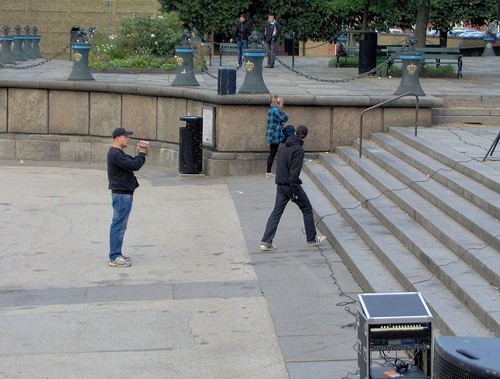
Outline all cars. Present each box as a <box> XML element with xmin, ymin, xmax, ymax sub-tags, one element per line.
<box><xmin>466</xmin><ymin>32</ymin><xmax>484</xmax><ymax>36</ymax></box>
<box><xmin>427</xmin><ymin>30</ymin><xmax>440</xmax><ymax>36</ymax></box>
<box><xmin>448</xmin><ymin>26</ymin><xmax>478</xmax><ymax>36</ymax></box>
<box><xmin>389</xmin><ymin>28</ymin><xmax>402</xmax><ymax>34</ymax></box>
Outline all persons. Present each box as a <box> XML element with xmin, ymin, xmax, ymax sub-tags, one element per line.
<box><xmin>264</xmin><ymin>14</ymin><xmax>281</xmax><ymax>69</ymax></box>
<box><xmin>336</xmin><ymin>41</ymin><xmax>348</xmax><ymax>66</ymax></box>
<box><xmin>260</xmin><ymin>125</ymin><xmax>327</xmax><ymax>250</ymax></box>
<box><xmin>229</xmin><ymin>14</ymin><xmax>251</xmax><ymax>67</ymax></box>
<box><xmin>265</xmin><ymin>95</ymin><xmax>289</xmax><ymax>178</ymax></box>
<box><xmin>107</xmin><ymin>128</ymin><xmax>150</xmax><ymax>267</ymax></box>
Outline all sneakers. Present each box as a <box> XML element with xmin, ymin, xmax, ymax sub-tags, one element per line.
<box><xmin>308</xmin><ymin>236</ymin><xmax>326</xmax><ymax>246</ymax></box>
<box><xmin>109</xmin><ymin>253</ymin><xmax>130</xmax><ymax>261</ymax></box>
<box><xmin>109</xmin><ymin>255</ymin><xmax>130</xmax><ymax>267</ymax></box>
<box><xmin>260</xmin><ymin>242</ymin><xmax>277</xmax><ymax>250</ymax></box>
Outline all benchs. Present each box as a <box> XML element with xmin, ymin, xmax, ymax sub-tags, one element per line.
<box><xmin>386</xmin><ymin>46</ymin><xmax>462</xmax><ymax>79</ymax></box>
<box><xmin>220</xmin><ymin>43</ymin><xmax>266</xmax><ymax>53</ymax></box>
<box><xmin>343</xmin><ymin>46</ymin><xmax>359</xmax><ymax>56</ymax></box>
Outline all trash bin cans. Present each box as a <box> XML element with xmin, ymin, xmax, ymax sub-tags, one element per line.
<box><xmin>358</xmin><ymin>32</ymin><xmax>378</xmax><ymax>76</ymax></box>
<box><xmin>178</xmin><ymin>115</ymin><xmax>203</xmax><ymax>174</ymax></box>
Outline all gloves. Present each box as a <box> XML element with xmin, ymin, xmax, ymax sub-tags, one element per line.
<box><xmin>291</xmin><ymin>191</ymin><xmax>299</xmax><ymax>202</ymax></box>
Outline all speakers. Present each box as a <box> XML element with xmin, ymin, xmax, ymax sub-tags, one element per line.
<box><xmin>431</xmin><ymin>336</ymin><xmax>500</xmax><ymax>379</ymax></box>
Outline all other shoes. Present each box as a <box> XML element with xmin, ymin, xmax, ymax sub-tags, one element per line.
<box><xmin>266</xmin><ymin>172</ymin><xmax>275</xmax><ymax>178</ymax></box>
<box><xmin>264</xmin><ymin>65</ymin><xmax>274</xmax><ymax>69</ymax></box>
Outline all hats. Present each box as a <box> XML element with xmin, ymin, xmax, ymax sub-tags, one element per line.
<box><xmin>112</xmin><ymin>128</ymin><xmax>133</xmax><ymax>139</ymax></box>
